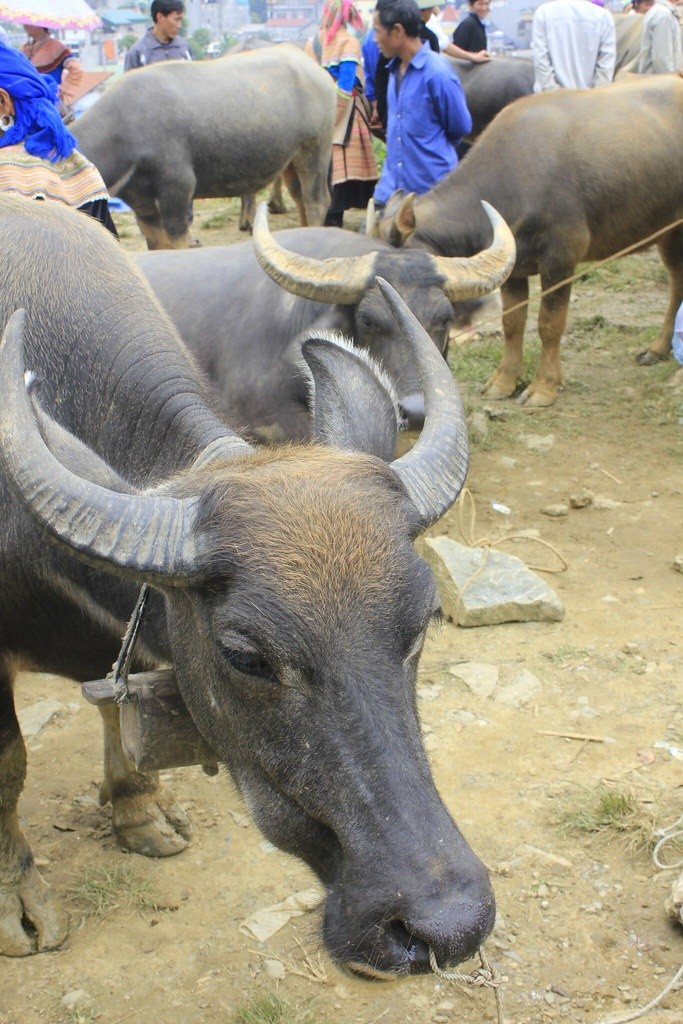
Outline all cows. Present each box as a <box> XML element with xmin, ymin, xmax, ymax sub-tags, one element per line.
<box><xmin>0</xmin><ymin>42</ymin><xmax>683</xmax><ymax>984</ymax></box>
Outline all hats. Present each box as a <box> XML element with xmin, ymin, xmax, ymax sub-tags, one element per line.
<box><xmin>415</xmin><ymin>0</ymin><xmax>446</xmax><ymax>10</ymax></box>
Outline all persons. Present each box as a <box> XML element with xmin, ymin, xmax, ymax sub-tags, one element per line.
<box><xmin>639</xmin><ymin>0</ymin><xmax>683</xmax><ymax>74</ymax></box>
<box><xmin>453</xmin><ymin>0</ymin><xmax>493</xmax><ymax>53</ymax></box>
<box><xmin>359</xmin><ymin>0</ymin><xmax>472</xmax><ymax>235</ymax></box>
<box><xmin>306</xmin><ymin>0</ymin><xmax>378</xmax><ymax>227</ymax></box>
<box><xmin>20</xmin><ymin>24</ymin><xmax>84</xmax><ymax>116</ymax></box>
<box><xmin>624</xmin><ymin>0</ymin><xmax>654</xmax><ymax>14</ymax></box>
<box><xmin>533</xmin><ymin>0</ymin><xmax>616</xmax><ymax>91</ymax></box>
<box><xmin>361</xmin><ymin>0</ymin><xmax>493</xmax><ymax>137</ymax></box>
<box><xmin>0</xmin><ymin>41</ymin><xmax>120</xmax><ymax>242</ymax></box>
<box><xmin>124</xmin><ymin>0</ymin><xmax>193</xmax><ymax>72</ymax></box>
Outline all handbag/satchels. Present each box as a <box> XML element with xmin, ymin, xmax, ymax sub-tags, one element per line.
<box><xmin>333</xmin><ymin>96</ymin><xmax>355</xmax><ymax>147</ymax></box>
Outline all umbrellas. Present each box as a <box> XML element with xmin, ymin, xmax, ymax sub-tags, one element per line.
<box><xmin>0</xmin><ymin>1</ymin><xmax>104</xmax><ymax>44</ymax></box>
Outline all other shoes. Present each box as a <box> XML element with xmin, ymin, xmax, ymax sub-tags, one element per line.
<box><xmin>447</xmin><ymin>328</ymin><xmax>483</xmax><ymax>349</ymax></box>
